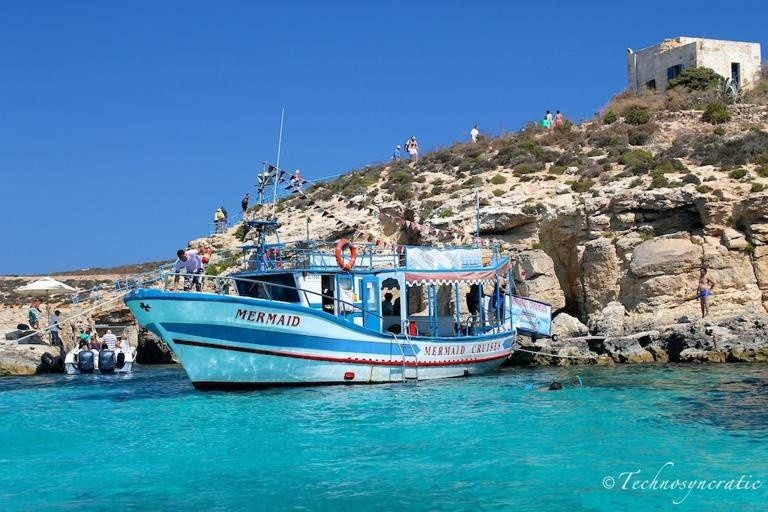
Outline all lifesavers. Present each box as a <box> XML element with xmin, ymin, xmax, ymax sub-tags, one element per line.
<box><xmin>335</xmin><ymin>239</ymin><xmax>356</xmax><ymax>270</ymax></box>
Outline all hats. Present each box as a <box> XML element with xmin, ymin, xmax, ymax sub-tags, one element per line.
<box><xmin>217</xmin><ymin>209</ymin><xmax>221</xmax><ymax>211</ymax></box>
<box><xmin>397</xmin><ymin>145</ymin><xmax>400</xmax><ymax>148</ymax></box>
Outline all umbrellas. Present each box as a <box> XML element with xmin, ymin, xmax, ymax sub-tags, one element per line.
<box><xmin>11</xmin><ymin>276</ymin><xmax>79</xmax><ymax>309</ymax></box>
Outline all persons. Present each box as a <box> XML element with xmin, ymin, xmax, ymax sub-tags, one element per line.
<box><xmin>465</xmin><ymin>284</ymin><xmax>487</xmax><ymax>334</ymax></box>
<box><xmin>240</xmin><ymin>193</ymin><xmax>250</xmax><ymax>211</ymax></box>
<box><xmin>214</xmin><ymin>209</ymin><xmax>220</xmax><ymax>234</ymax></box>
<box><xmin>48</xmin><ymin>310</ymin><xmax>62</xmax><ymax>346</ymax></box>
<box><xmin>553</xmin><ymin>110</ymin><xmax>563</xmax><ymax>126</ymax></box>
<box><xmin>470</xmin><ymin>125</ymin><xmax>479</xmax><ymax>143</ymax></box>
<box><xmin>219</xmin><ymin>205</ymin><xmax>227</xmax><ymax>234</ymax></box>
<box><xmin>544</xmin><ymin>110</ymin><xmax>553</xmax><ymax>121</ymax></box>
<box><xmin>380</xmin><ymin>292</ymin><xmax>393</xmax><ymax>317</ymax></box>
<box><xmin>539</xmin><ymin>116</ymin><xmax>550</xmax><ymax>130</ymax></box>
<box><xmin>393</xmin><ymin>145</ymin><xmax>401</xmax><ymax>159</ymax></box>
<box><xmin>71</xmin><ymin>325</ymin><xmax>130</xmax><ymax>368</ymax></box>
<box><xmin>173</xmin><ymin>247</ymin><xmax>214</xmax><ymax>292</ymax></box>
<box><xmin>487</xmin><ymin>271</ymin><xmax>509</xmax><ymax>332</ymax></box>
<box><xmin>405</xmin><ymin>136</ymin><xmax>421</xmax><ymax>161</ymax></box>
<box><xmin>534</xmin><ymin>380</ymin><xmax>564</xmax><ymax>394</ymax></box>
<box><xmin>698</xmin><ymin>268</ymin><xmax>715</xmax><ymax>318</ymax></box>
<box><xmin>28</xmin><ymin>299</ymin><xmax>42</xmax><ymax>331</ymax></box>
<box><xmin>290</xmin><ymin>170</ymin><xmax>303</xmax><ymax>190</ymax></box>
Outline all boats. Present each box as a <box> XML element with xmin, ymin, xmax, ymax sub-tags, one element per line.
<box><xmin>63</xmin><ymin>325</ymin><xmax>138</xmax><ymax>375</ymax></box>
<box><xmin>120</xmin><ymin>108</ymin><xmax>517</xmax><ymax>391</ymax></box>
